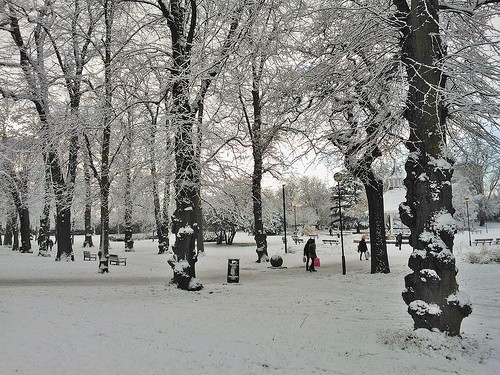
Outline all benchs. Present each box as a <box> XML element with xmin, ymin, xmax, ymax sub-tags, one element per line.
<box><xmin>494</xmin><ymin>238</ymin><xmax>500</xmax><ymax>244</ymax></box>
<box><xmin>307</xmin><ymin>234</ymin><xmax>319</xmax><ymax>239</ymax></box>
<box><xmin>108</xmin><ymin>254</ymin><xmax>127</xmax><ymax>266</ymax></box>
<box><xmin>471</xmin><ymin>239</ymin><xmax>494</xmax><ymax>245</ymax></box>
<box><xmin>84</xmin><ymin>251</ymin><xmax>97</xmax><ymax>262</ymax></box>
<box><xmin>291</xmin><ymin>235</ymin><xmax>304</xmax><ymax>244</ymax></box>
<box><xmin>321</xmin><ymin>240</ymin><xmax>340</xmax><ymax>245</ymax></box>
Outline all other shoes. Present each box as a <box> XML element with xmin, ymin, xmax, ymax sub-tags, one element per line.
<box><xmin>310</xmin><ymin>268</ymin><xmax>317</xmax><ymax>272</ymax></box>
<box><xmin>306</xmin><ymin>268</ymin><xmax>310</xmax><ymax>271</ymax></box>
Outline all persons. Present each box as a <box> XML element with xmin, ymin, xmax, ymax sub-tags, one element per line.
<box><xmin>396</xmin><ymin>233</ymin><xmax>403</xmax><ymax>250</ymax></box>
<box><xmin>358</xmin><ymin>238</ymin><xmax>369</xmax><ymax>260</ymax></box>
<box><xmin>38</xmin><ymin>236</ymin><xmax>54</xmax><ymax>251</ymax></box>
<box><xmin>303</xmin><ymin>238</ymin><xmax>319</xmax><ymax>273</ymax></box>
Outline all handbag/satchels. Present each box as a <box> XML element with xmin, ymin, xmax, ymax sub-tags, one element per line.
<box><xmin>365</xmin><ymin>250</ymin><xmax>371</xmax><ymax>258</ymax></box>
<box><xmin>314</xmin><ymin>256</ymin><xmax>320</xmax><ymax>266</ymax></box>
<box><xmin>395</xmin><ymin>242</ymin><xmax>398</xmax><ymax>246</ymax></box>
